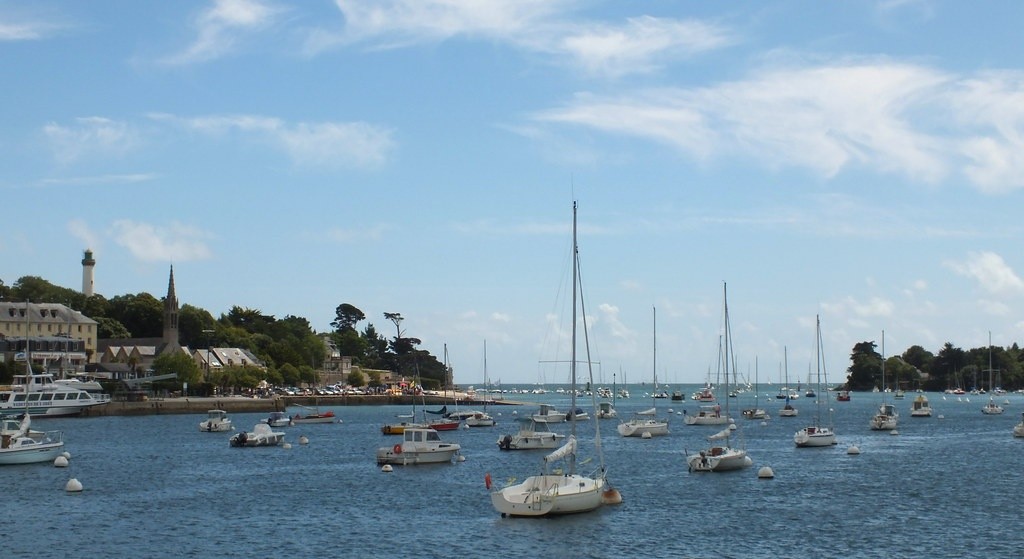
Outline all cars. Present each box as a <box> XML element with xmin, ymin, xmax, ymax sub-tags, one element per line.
<box><xmin>242</xmin><ymin>385</ymin><xmax>368</xmax><ymax>396</ymax></box>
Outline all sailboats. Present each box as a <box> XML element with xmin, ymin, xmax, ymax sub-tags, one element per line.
<box><xmin>379</xmin><ymin>341</ymin><xmax>501</xmax><ymax>435</ymax></box>
<box><xmin>869</xmin><ymin>331</ymin><xmax>900</xmax><ymax>431</ymax></box>
<box><xmin>487</xmin><ymin>201</ymin><xmax>623</xmax><ymax>518</ymax></box>
<box><xmin>636</xmin><ymin>283</ymin><xmax>851</xmax><ymax>474</ymax></box>
<box><xmin>0</xmin><ymin>298</ymin><xmax>65</xmax><ymax>465</ymax></box>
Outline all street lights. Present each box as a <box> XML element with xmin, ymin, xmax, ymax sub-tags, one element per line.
<box><xmin>303</xmin><ymin>347</ymin><xmax>316</xmax><ymax>394</ymax></box>
<box><xmin>202</xmin><ymin>330</ymin><xmax>216</xmax><ymax>397</ymax></box>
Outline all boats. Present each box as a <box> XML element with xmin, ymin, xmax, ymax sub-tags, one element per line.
<box><xmin>377</xmin><ymin>428</ymin><xmax>461</xmax><ymax>466</ymax></box>
<box><xmin>496</xmin><ymin>404</ymin><xmax>568</xmax><ymax>451</ymax></box>
<box><xmin>199</xmin><ymin>410</ymin><xmax>235</xmax><ymax>432</ymax></box>
<box><xmin>896</xmin><ymin>332</ymin><xmax>1023</xmax><ymax>437</ymax></box>
<box><xmin>0</xmin><ymin>372</ymin><xmax>111</xmax><ymax>419</ymax></box>
<box><xmin>618</xmin><ymin>419</ymin><xmax>667</xmax><ymax>438</ymax></box>
<box><xmin>260</xmin><ymin>411</ymin><xmax>291</xmax><ymax>426</ymax></box>
<box><xmin>230</xmin><ymin>423</ymin><xmax>286</xmax><ymax>447</ymax></box>
<box><xmin>291</xmin><ymin>411</ymin><xmax>336</xmax><ymax>423</ymax></box>
<box><xmin>566</xmin><ymin>402</ymin><xmax>617</xmax><ymax>421</ymax></box>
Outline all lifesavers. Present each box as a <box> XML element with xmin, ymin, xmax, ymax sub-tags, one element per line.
<box><xmin>743</xmin><ymin>411</ymin><xmax>747</xmax><ymax>414</ymax></box>
<box><xmin>394</xmin><ymin>444</ymin><xmax>401</xmax><ymax>453</ymax></box>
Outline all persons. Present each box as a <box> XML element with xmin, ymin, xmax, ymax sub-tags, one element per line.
<box><xmin>715</xmin><ymin>403</ymin><xmax>721</xmax><ymax>418</ymax></box>
<box><xmin>918</xmin><ymin>395</ymin><xmax>923</xmax><ymax>401</ymax></box>
<box><xmin>367</xmin><ymin>384</ymin><xmax>388</xmax><ymax>395</ymax></box>
<box><xmin>797</xmin><ymin>381</ymin><xmax>801</xmax><ymax>391</ymax></box>
<box><xmin>876</xmin><ymin>404</ymin><xmax>887</xmax><ymax>416</ymax></box>
